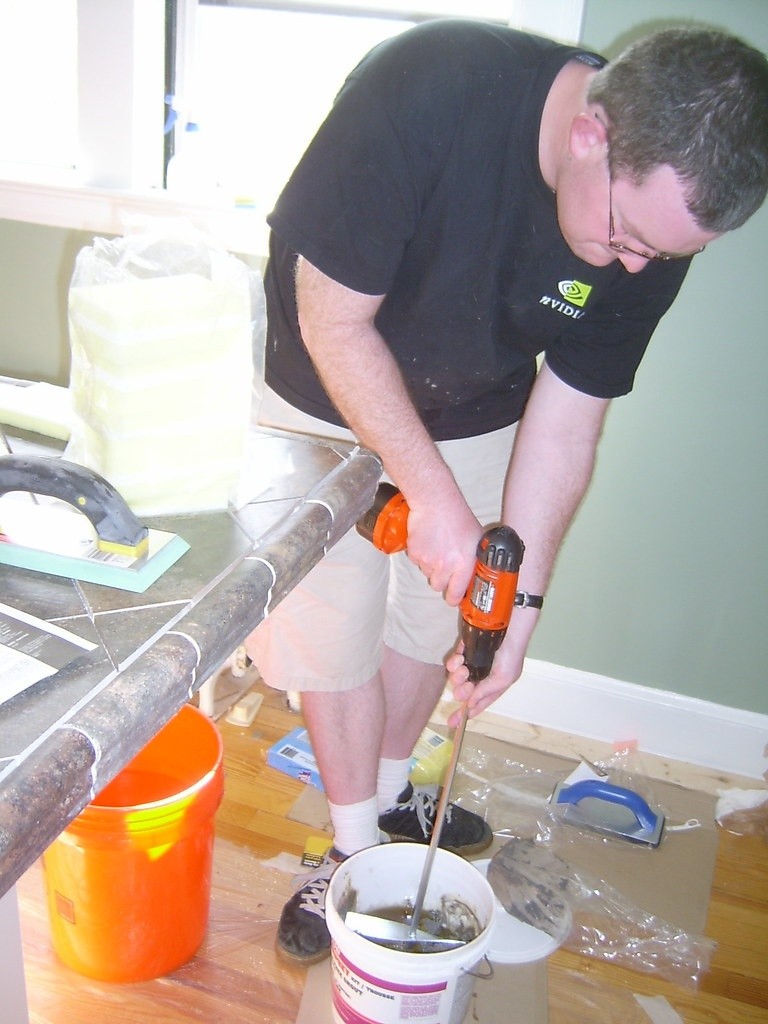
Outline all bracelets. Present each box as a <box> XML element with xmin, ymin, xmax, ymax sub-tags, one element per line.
<box><xmin>512</xmin><ymin>591</ymin><xmax>543</xmax><ymax>609</ymax></box>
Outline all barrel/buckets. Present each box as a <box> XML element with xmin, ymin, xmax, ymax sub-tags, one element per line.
<box><xmin>42</xmin><ymin>708</ymin><xmax>223</xmax><ymax>982</ymax></box>
<box><xmin>325</xmin><ymin>842</ymin><xmax>495</xmax><ymax>1024</ymax></box>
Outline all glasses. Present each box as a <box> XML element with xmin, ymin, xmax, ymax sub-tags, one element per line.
<box><xmin>595</xmin><ymin>114</ymin><xmax>705</xmax><ymax>262</ymax></box>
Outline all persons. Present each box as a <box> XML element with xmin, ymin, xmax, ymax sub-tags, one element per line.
<box><xmin>231</xmin><ymin>21</ymin><xmax>768</xmax><ymax>970</ymax></box>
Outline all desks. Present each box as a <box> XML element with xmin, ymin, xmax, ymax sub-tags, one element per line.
<box><xmin>0</xmin><ymin>376</ymin><xmax>385</xmax><ymax>1024</ymax></box>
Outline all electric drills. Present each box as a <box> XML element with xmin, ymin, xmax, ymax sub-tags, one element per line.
<box><xmin>342</xmin><ymin>483</ymin><xmax>527</xmax><ymax>947</ymax></box>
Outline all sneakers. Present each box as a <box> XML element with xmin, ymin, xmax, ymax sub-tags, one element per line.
<box><xmin>276</xmin><ymin>831</ymin><xmax>390</xmax><ymax>967</ymax></box>
<box><xmin>378</xmin><ymin>780</ymin><xmax>493</xmax><ymax>855</ymax></box>
